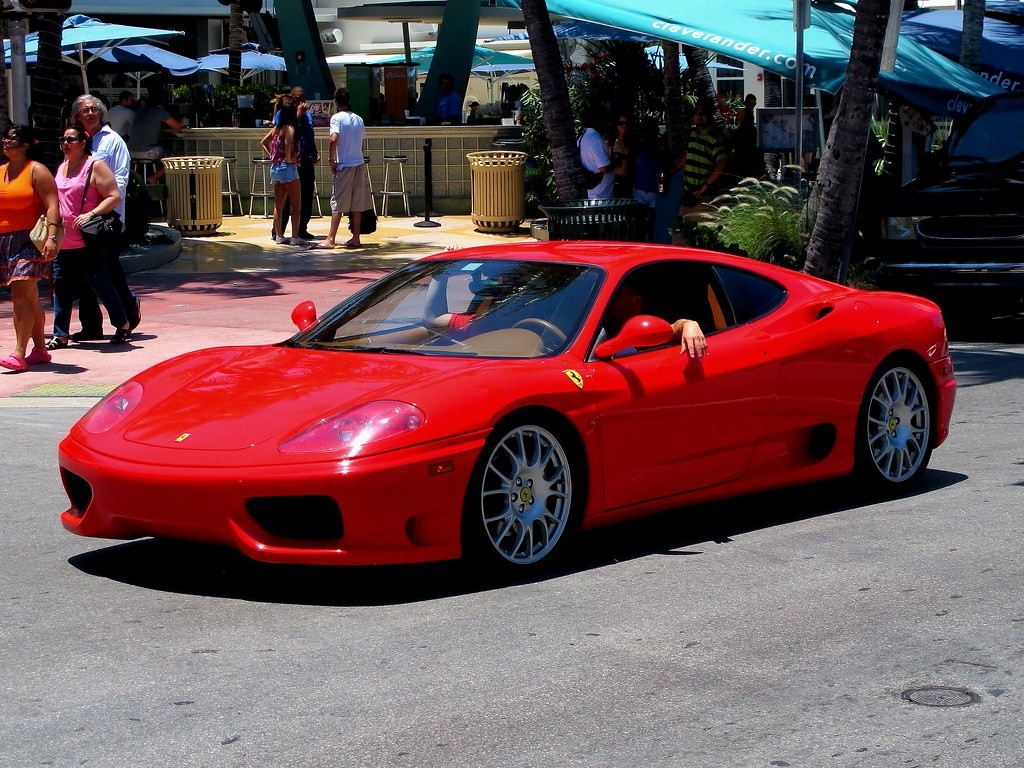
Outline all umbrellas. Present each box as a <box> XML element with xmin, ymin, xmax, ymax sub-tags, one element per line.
<box><xmin>196</xmin><ymin>43</ymin><xmax>287</xmax><ymax>85</ymax></box>
<box><xmin>71</xmin><ymin>45</ymin><xmax>202</xmax><ymax>101</ymax></box>
<box><xmin>5</xmin><ymin>14</ymin><xmax>185</xmax><ymax>94</ymax></box>
<box><xmin>899</xmin><ymin>10</ymin><xmax>1024</xmax><ymax>92</ymax></box>
<box><xmin>368</xmin><ymin>44</ymin><xmax>535</xmax><ymax>102</ymax></box>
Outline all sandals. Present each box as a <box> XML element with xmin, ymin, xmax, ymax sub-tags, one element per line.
<box><xmin>314</xmin><ymin>242</ymin><xmax>334</xmax><ymax>249</ymax></box>
<box><xmin>46</xmin><ymin>337</ymin><xmax>67</xmax><ymax>350</ymax></box>
<box><xmin>341</xmin><ymin>239</ymin><xmax>362</xmax><ymax>248</ymax></box>
<box><xmin>0</xmin><ymin>354</ymin><xmax>28</xmax><ymax>370</ymax></box>
<box><xmin>109</xmin><ymin>324</ymin><xmax>131</xmax><ymax>343</ymax></box>
<box><xmin>25</xmin><ymin>351</ymin><xmax>52</xmax><ymax>364</ymax></box>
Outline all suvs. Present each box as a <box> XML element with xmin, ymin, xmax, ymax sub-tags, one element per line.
<box><xmin>863</xmin><ymin>89</ymin><xmax>1024</xmax><ymax>346</ymax></box>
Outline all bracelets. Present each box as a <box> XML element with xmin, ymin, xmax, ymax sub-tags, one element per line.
<box><xmin>48</xmin><ymin>224</ymin><xmax>57</xmax><ymax>226</ymax></box>
<box><xmin>704</xmin><ymin>182</ymin><xmax>709</xmax><ymax>185</ymax></box>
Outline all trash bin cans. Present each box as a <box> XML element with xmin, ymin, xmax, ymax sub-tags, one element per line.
<box><xmin>466</xmin><ymin>150</ymin><xmax>529</xmax><ymax>233</ymax></box>
<box><xmin>538</xmin><ymin>197</ymin><xmax>641</xmax><ymax>241</ymax></box>
<box><xmin>160</xmin><ymin>156</ymin><xmax>226</xmax><ymax>237</ymax></box>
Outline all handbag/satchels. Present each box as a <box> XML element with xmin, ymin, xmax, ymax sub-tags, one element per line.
<box><xmin>79</xmin><ymin>210</ymin><xmax>123</xmax><ymax>268</ymax></box>
<box><xmin>585</xmin><ymin>167</ymin><xmax>603</xmax><ymax>190</ymax></box>
<box><xmin>348</xmin><ymin>210</ymin><xmax>376</xmax><ymax>234</ymax></box>
<box><xmin>29</xmin><ymin>215</ymin><xmax>65</xmax><ymax>261</ymax></box>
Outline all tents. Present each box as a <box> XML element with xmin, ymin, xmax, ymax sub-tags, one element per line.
<box><xmin>541</xmin><ymin>0</ymin><xmax>1009</xmax><ymax>160</ymax></box>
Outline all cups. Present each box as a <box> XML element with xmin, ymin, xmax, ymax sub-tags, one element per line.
<box><xmin>256</xmin><ymin>120</ymin><xmax>261</xmax><ymax>127</ymax></box>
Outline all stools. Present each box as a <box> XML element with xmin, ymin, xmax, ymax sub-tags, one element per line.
<box><xmin>132</xmin><ymin>159</ymin><xmax>165</xmax><ymax>217</ymax></box>
<box><xmin>221</xmin><ymin>155</ymin><xmax>412</xmax><ymax>218</ymax></box>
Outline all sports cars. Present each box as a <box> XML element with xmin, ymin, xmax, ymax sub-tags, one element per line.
<box><xmin>56</xmin><ymin>242</ymin><xmax>956</xmax><ymax>589</ymax></box>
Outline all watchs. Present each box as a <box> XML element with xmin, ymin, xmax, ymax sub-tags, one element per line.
<box><xmin>48</xmin><ymin>234</ymin><xmax>57</xmax><ymax>241</ymax></box>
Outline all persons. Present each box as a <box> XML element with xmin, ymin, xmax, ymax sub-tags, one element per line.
<box><xmin>106</xmin><ymin>90</ymin><xmax>136</xmax><ymax>147</ymax></box>
<box><xmin>577</xmin><ymin>103</ymin><xmax>665</xmax><ymax>204</ymax></box>
<box><xmin>421</xmin><ymin>246</ymin><xmax>484</xmax><ymax>337</ymax></box>
<box><xmin>0</xmin><ymin>124</ymin><xmax>58</xmax><ymax>371</ymax></box>
<box><xmin>588</xmin><ymin>276</ymin><xmax>709</xmax><ymax>359</ymax></box>
<box><xmin>432</xmin><ymin>74</ymin><xmax>461</xmax><ymax>119</ymax></box>
<box><xmin>127</xmin><ymin>89</ymin><xmax>187</xmax><ymax>185</ymax></box>
<box><xmin>468</xmin><ymin>102</ymin><xmax>479</xmax><ymax>118</ymax></box>
<box><xmin>44</xmin><ymin>126</ymin><xmax>131</xmax><ymax>353</ymax></box>
<box><xmin>312</xmin><ymin>88</ymin><xmax>367</xmax><ymax>249</ymax></box>
<box><xmin>260</xmin><ymin>87</ymin><xmax>320</xmax><ymax>245</ymax></box>
<box><xmin>668</xmin><ymin>93</ymin><xmax>756</xmax><ymax>214</ymax></box>
<box><xmin>68</xmin><ymin>95</ymin><xmax>130</xmax><ymax>342</ymax></box>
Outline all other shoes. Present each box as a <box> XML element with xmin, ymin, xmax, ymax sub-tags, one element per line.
<box><xmin>275</xmin><ymin>236</ymin><xmax>290</xmax><ymax>244</ymax></box>
<box><xmin>72</xmin><ymin>329</ymin><xmax>103</xmax><ymax>340</ymax></box>
<box><xmin>298</xmin><ymin>232</ymin><xmax>315</xmax><ymax>238</ymax></box>
<box><xmin>130</xmin><ymin>298</ymin><xmax>141</xmax><ymax>328</ymax></box>
<box><xmin>272</xmin><ymin>232</ymin><xmax>276</xmax><ymax>240</ymax></box>
<box><xmin>291</xmin><ymin>237</ymin><xmax>310</xmax><ymax>245</ymax></box>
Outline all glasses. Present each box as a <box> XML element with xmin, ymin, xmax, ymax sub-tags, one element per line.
<box><xmin>3</xmin><ymin>135</ymin><xmax>20</xmax><ymax>141</ymax></box>
<box><xmin>619</xmin><ymin>121</ymin><xmax>630</xmax><ymax>126</ymax></box>
<box><xmin>693</xmin><ymin>109</ymin><xmax>707</xmax><ymax>116</ymax></box>
<box><xmin>59</xmin><ymin>137</ymin><xmax>80</xmax><ymax>142</ymax></box>
